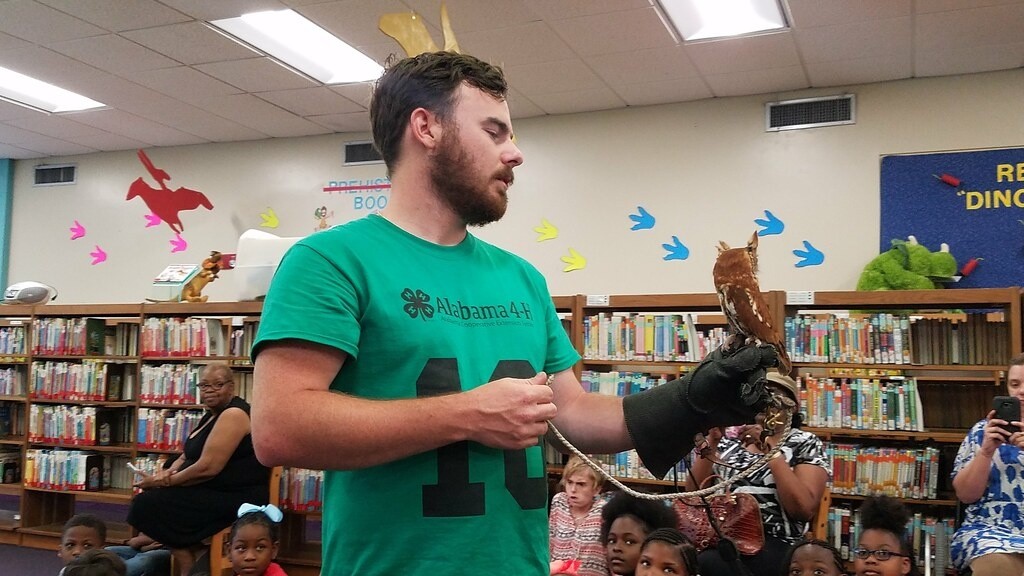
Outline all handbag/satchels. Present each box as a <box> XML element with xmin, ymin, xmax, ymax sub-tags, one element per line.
<box><xmin>672</xmin><ymin>475</ymin><xmax>765</xmax><ymax>554</ymax></box>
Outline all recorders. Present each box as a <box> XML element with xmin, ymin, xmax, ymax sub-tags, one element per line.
<box><xmin>2</xmin><ymin>281</ymin><xmax>58</xmax><ymax>305</ymax></box>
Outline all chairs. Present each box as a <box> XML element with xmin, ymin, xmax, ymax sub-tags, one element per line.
<box><xmin>170</xmin><ymin>463</ymin><xmax>281</xmax><ymax>576</ymax></box>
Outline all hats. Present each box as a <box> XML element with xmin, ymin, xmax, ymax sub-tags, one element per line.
<box><xmin>763</xmin><ymin>370</ymin><xmax>798</xmax><ymax>405</ymax></box>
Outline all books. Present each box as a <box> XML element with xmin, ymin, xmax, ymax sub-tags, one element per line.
<box><xmin>279</xmin><ymin>465</ymin><xmax>324</xmax><ymax>511</ymax></box>
<box><xmin>796</xmin><ymin>367</ymin><xmax>1006</xmax><ymax>430</ymax></box>
<box><xmin>546</xmin><ymin>366</ymin><xmax>695</xmax><ymax>483</ymax></box>
<box><xmin>584</xmin><ymin>312</ymin><xmax>727</xmax><ymax>363</ymax></box>
<box><xmin>828</xmin><ymin>503</ymin><xmax>961</xmax><ymax>569</ymax></box>
<box><xmin>782</xmin><ymin>311</ymin><xmax>1013</xmax><ymax>365</ymax></box>
<box><xmin>822</xmin><ymin>441</ymin><xmax>957</xmax><ymax>500</ymax></box>
<box><xmin>0</xmin><ymin>317</ymin><xmax>260</xmax><ymax>491</ymax></box>
<box><xmin>154</xmin><ymin>264</ymin><xmax>200</xmax><ymax>286</ymax></box>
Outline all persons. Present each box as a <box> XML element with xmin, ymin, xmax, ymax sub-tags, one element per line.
<box><xmin>636</xmin><ymin>528</ymin><xmax>698</xmax><ymax>576</ymax></box>
<box><xmin>599</xmin><ymin>492</ymin><xmax>676</xmax><ymax>576</ymax></box>
<box><xmin>550</xmin><ymin>454</ymin><xmax>609</xmax><ymax>576</ymax></box>
<box><xmin>948</xmin><ymin>353</ymin><xmax>1024</xmax><ymax>576</ymax></box>
<box><xmin>226</xmin><ymin>503</ymin><xmax>288</xmax><ymax>576</ymax></box>
<box><xmin>249</xmin><ymin>51</ymin><xmax>782</xmax><ymax>576</ymax></box>
<box><xmin>127</xmin><ymin>362</ymin><xmax>261</xmax><ymax>576</ymax></box>
<box><xmin>783</xmin><ymin>539</ymin><xmax>850</xmax><ymax>576</ymax></box>
<box><xmin>855</xmin><ymin>495</ymin><xmax>921</xmax><ymax>576</ymax></box>
<box><xmin>57</xmin><ymin>513</ymin><xmax>172</xmax><ymax>576</ymax></box>
<box><xmin>685</xmin><ymin>374</ymin><xmax>833</xmax><ymax>576</ymax></box>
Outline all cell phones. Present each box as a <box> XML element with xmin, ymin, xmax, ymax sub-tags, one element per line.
<box><xmin>993</xmin><ymin>395</ymin><xmax>1021</xmax><ymax>441</ymax></box>
<box><xmin>723</xmin><ymin>423</ymin><xmax>745</xmax><ymax>439</ymax></box>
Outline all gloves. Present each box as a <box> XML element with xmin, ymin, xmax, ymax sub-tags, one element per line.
<box><xmin>623</xmin><ymin>343</ymin><xmax>779</xmax><ymax>481</ymax></box>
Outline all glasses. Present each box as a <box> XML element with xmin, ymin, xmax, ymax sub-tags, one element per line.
<box><xmin>852</xmin><ymin>547</ymin><xmax>907</xmax><ymax>560</ymax></box>
<box><xmin>196</xmin><ymin>379</ymin><xmax>232</xmax><ymax>391</ymax></box>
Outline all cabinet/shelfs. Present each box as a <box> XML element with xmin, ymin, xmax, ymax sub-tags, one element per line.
<box><xmin>1</xmin><ymin>285</ymin><xmax>1024</xmax><ymax>576</ymax></box>
